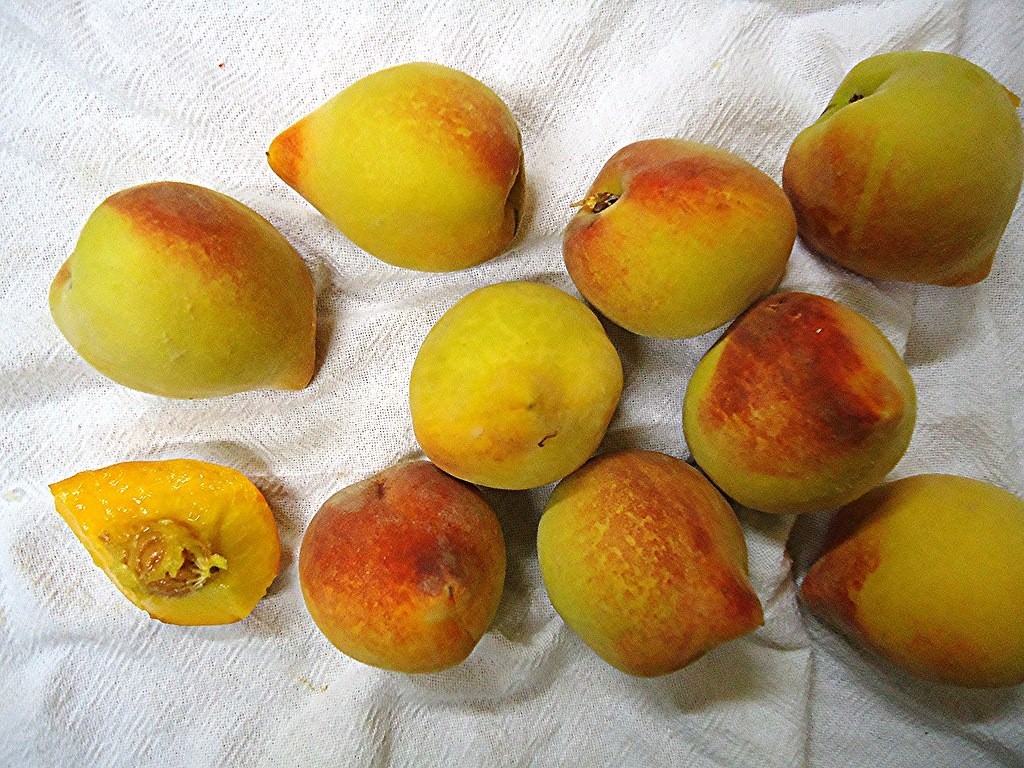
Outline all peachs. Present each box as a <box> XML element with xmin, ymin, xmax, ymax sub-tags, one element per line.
<box><xmin>47</xmin><ymin>51</ymin><xmax>1023</xmax><ymax>687</ymax></box>
